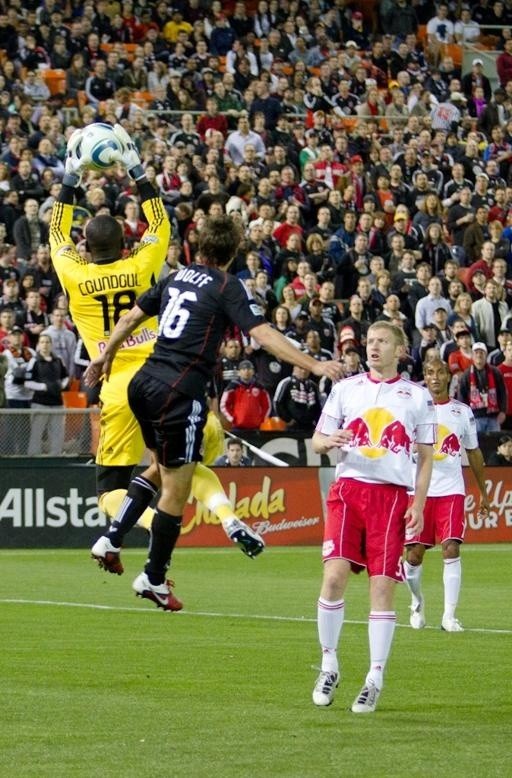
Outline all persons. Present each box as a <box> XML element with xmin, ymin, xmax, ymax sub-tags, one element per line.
<box><xmin>306</xmin><ymin>319</ymin><xmax>438</xmax><ymax>714</ymax></box>
<box><xmin>48</xmin><ymin>121</ymin><xmax>266</xmax><ymax>561</ymax></box>
<box><xmin>402</xmin><ymin>356</ymin><xmax>493</xmax><ymax>632</ymax></box>
<box><xmin>1</xmin><ymin>1</ymin><xmax>512</xmax><ymax>465</ymax></box>
<box><xmin>82</xmin><ymin>212</ymin><xmax>346</xmax><ymax>612</ymax></box>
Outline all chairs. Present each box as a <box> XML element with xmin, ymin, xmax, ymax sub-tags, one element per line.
<box><xmin>60</xmin><ymin>391</ymin><xmax>88</xmax><ymax>441</ymax></box>
<box><xmin>129</xmin><ymin>93</ymin><xmax>153</xmax><ymax>109</ymax></box>
<box><xmin>218</xmin><ymin>57</ymin><xmax>229</xmax><ymax>72</ymax></box>
<box><xmin>123</xmin><ymin>43</ymin><xmax>137</xmax><ymax>62</ymax></box>
<box><xmin>261</xmin><ymin>417</ymin><xmax>287</xmax><ymax>433</ymax></box>
<box><xmin>418</xmin><ymin>25</ymin><xmax>486</xmax><ymax>66</ymax></box>
<box><xmin>42</xmin><ymin>70</ymin><xmax>67</xmax><ymax>97</ymax></box>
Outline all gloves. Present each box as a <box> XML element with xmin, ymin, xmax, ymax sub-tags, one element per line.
<box><xmin>111</xmin><ymin>123</ymin><xmax>146</xmax><ymax>181</ymax></box>
<box><xmin>62</xmin><ymin>129</ymin><xmax>92</xmax><ymax>189</ymax></box>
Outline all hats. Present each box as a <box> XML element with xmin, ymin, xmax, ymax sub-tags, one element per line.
<box><xmin>472</xmin><ymin>342</ymin><xmax>486</xmax><ymax>352</ymax></box>
<box><xmin>238</xmin><ymin>360</ymin><xmax>253</xmax><ymax>369</ymax></box>
<box><xmin>456</xmin><ymin>329</ymin><xmax>469</xmax><ymax>337</ymax></box>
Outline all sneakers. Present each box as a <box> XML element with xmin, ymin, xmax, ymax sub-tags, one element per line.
<box><xmin>222</xmin><ymin>517</ymin><xmax>265</xmax><ymax>559</ymax></box>
<box><xmin>351</xmin><ymin>678</ymin><xmax>380</xmax><ymax>713</ymax></box>
<box><xmin>131</xmin><ymin>572</ymin><xmax>182</xmax><ymax>612</ymax></box>
<box><xmin>441</xmin><ymin>619</ymin><xmax>464</xmax><ymax>632</ymax></box>
<box><xmin>408</xmin><ymin>601</ymin><xmax>426</xmax><ymax>629</ymax></box>
<box><xmin>311</xmin><ymin>665</ymin><xmax>340</xmax><ymax>706</ymax></box>
<box><xmin>91</xmin><ymin>536</ymin><xmax>124</xmax><ymax>575</ymax></box>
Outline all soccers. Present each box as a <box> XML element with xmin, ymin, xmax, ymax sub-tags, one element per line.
<box><xmin>77</xmin><ymin>122</ymin><xmax>123</xmax><ymax>171</ymax></box>
